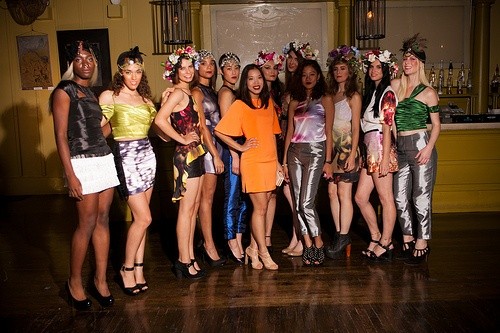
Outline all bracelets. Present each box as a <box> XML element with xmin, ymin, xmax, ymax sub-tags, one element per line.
<box><xmin>325</xmin><ymin>161</ymin><xmax>332</xmax><ymax>164</ymax></box>
<box><xmin>282</xmin><ymin>164</ymin><xmax>288</xmax><ymax>166</ymax></box>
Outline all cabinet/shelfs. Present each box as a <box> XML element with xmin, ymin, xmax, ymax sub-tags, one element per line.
<box><xmin>423</xmin><ymin>68</ymin><xmax>475</xmax><ymax>116</ymax></box>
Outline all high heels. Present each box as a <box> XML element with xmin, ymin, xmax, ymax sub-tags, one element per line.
<box><xmin>134</xmin><ymin>263</ymin><xmax>149</xmax><ymax>292</ymax></box>
<box><xmin>326</xmin><ymin>234</ymin><xmax>352</xmax><ymax>257</ymax></box>
<box><xmin>89</xmin><ymin>277</ymin><xmax>113</xmax><ymax>307</ymax></box>
<box><xmin>223</xmin><ymin>239</ymin><xmax>246</xmax><ymax>264</ymax></box>
<box><xmin>265</xmin><ymin>236</ymin><xmax>273</xmax><ymax>252</ymax></box>
<box><xmin>405</xmin><ymin>245</ymin><xmax>430</xmax><ymax>264</ymax></box>
<box><xmin>362</xmin><ymin>241</ymin><xmax>394</xmax><ymax>260</ymax></box>
<box><xmin>360</xmin><ymin>240</ymin><xmax>379</xmax><ymax>257</ymax></box>
<box><xmin>200</xmin><ymin>243</ymin><xmax>225</xmax><ymax>267</ymax></box>
<box><xmin>191</xmin><ymin>259</ymin><xmax>207</xmax><ymax>273</ymax></box>
<box><xmin>258</xmin><ymin>251</ymin><xmax>278</xmax><ymax>269</ymax></box>
<box><xmin>64</xmin><ymin>281</ymin><xmax>92</xmax><ymax>311</ymax></box>
<box><xmin>326</xmin><ymin>232</ymin><xmax>340</xmax><ymax>252</ymax></box>
<box><xmin>400</xmin><ymin>239</ymin><xmax>416</xmax><ymax>258</ymax></box>
<box><xmin>245</xmin><ymin>246</ymin><xmax>263</xmax><ymax>269</ymax></box>
<box><xmin>175</xmin><ymin>259</ymin><xmax>207</xmax><ymax>280</ymax></box>
<box><xmin>113</xmin><ymin>265</ymin><xmax>140</xmax><ymax>296</ymax></box>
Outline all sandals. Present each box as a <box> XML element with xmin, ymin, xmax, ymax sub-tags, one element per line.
<box><xmin>303</xmin><ymin>244</ymin><xmax>314</xmax><ymax>267</ymax></box>
<box><xmin>313</xmin><ymin>244</ymin><xmax>325</xmax><ymax>267</ymax></box>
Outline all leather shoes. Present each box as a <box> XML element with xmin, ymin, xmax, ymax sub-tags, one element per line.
<box><xmin>282</xmin><ymin>241</ymin><xmax>299</xmax><ymax>253</ymax></box>
<box><xmin>288</xmin><ymin>248</ymin><xmax>303</xmax><ymax>256</ymax></box>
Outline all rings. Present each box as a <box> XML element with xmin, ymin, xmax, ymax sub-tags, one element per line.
<box><xmin>385</xmin><ymin>171</ymin><xmax>388</xmax><ymax>175</ymax></box>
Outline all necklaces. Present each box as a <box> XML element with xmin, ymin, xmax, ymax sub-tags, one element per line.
<box><xmin>335</xmin><ymin>92</ymin><xmax>346</xmax><ymax>100</ymax></box>
<box><xmin>252</xmin><ymin>97</ymin><xmax>260</xmax><ymax>109</ymax></box>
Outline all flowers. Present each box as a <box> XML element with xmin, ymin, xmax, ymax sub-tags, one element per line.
<box><xmin>161</xmin><ymin>45</ymin><xmax>200</xmax><ymax>83</ymax></box>
<box><xmin>325</xmin><ymin>45</ymin><xmax>361</xmax><ymax>65</ymax></box>
<box><xmin>357</xmin><ymin>48</ymin><xmax>397</xmax><ymax>77</ymax></box>
<box><xmin>284</xmin><ymin>32</ymin><xmax>320</xmax><ymax>61</ymax></box>
<box><xmin>255</xmin><ymin>50</ymin><xmax>285</xmax><ymax>69</ymax></box>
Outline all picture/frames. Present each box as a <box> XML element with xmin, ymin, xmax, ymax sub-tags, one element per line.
<box><xmin>56</xmin><ymin>29</ymin><xmax>113</xmax><ymax>96</ymax></box>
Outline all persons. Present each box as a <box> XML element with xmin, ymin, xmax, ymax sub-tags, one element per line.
<box><xmin>354</xmin><ymin>50</ymin><xmax>400</xmax><ymax>263</ymax></box>
<box><xmin>49</xmin><ymin>39</ymin><xmax>121</xmax><ymax>310</ymax></box>
<box><xmin>325</xmin><ymin>45</ymin><xmax>362</xmax><ymax>259</ymax></box>
<box><xmin>213</xmin><ymin>63</ymin><xmax>285</xmax><ymax>270</ymax></box>
<box><xmin>189</xmin><ymin>49</ymin><xmax>227</xmax><ymax>267</ymax></box>
<box><xmin>391</xmin><ymin>32</ymin><xmax>441</xmax><ymax>264</ymax></box>
<box><xmin>155</xmin><ymin>46</ymin><xmax>209</xmax><ymax>281</ymax></box>
<box><xmin>253</xmin><ymin>49</ymin><xmax>284</xmax><ymax>252</ymax></box>
<box><xmin>282</xmin><ymin>60</ymin><xmax>335</xmax><ymax>267</ymax></box>
<box><xmin>216</xmin><ymin>51</ymin><xmax>251</xmax><ymax>265</ymax></box>
<box><xmin>99</xmin><ymin>46</ymin><xmax>175</xmax><ymax>296</ymax></box>
<box><xmin>280</xmin><ymin>40</ymin><xmax>321</xmax><ymax>256</ymax></box>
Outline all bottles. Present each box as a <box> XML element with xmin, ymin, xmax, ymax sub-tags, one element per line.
<box><xmin>429</xmin><ymin>58</ymin><xmax>473</xmax><ymax>94</ymax></box>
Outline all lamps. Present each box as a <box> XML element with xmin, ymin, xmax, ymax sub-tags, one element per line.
<box><xmin>162</xmin><ymin>0</ymin><xmax>192</xmax><ymax>43</ymax></box>
<box><xmin>6</xmin><ymin>0</ymin><xmax>50</xmax><ymax>26</ymax></box>
<box><xmin>351</xmin><ymin>0</ymin><xmax>386</xmax><ymax>40</ymax></box>
